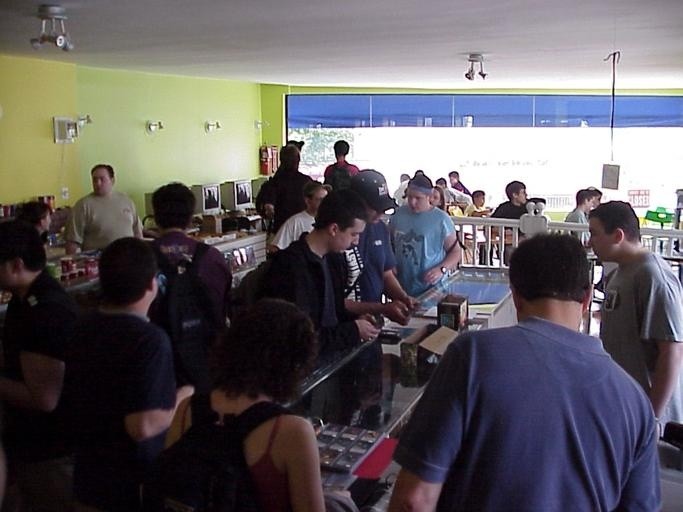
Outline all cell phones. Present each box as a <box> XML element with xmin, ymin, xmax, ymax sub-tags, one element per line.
<box><xmin>379</xmin><ymin>331</ymin><xmax>401</xmax><ymax>339</ymax></box>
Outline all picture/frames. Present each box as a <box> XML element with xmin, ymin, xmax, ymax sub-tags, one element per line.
<box><xmin>54</xmin><ymin>116</ymin><xmax>74</xmax><ymax>144</ymax></box>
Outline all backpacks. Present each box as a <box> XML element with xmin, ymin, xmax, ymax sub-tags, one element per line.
<box><xmin>142</xmin><ymin>392</ymin><xmax>298</xmax><ymax>511</ymax></box>
<box><xmin>326</xmin><ymin>164</ymin><xmax>352</xmax><ymax>193</ymax></box>
<box><xmin>152</xmin><ymin>241</ymin><xmax>227</xmax><ymax>386</ymax></box>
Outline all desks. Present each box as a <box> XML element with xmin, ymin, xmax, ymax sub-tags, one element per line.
<box><xmin>463</xmin><ymin>229</ymin><xmax>515</xmax><ymax>264</ymax></box>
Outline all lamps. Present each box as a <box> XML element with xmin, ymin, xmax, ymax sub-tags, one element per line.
<box><xmin>205</xmin><ymin>119</ymin><xmax>222</xmax><ymax>132</ymax></box>
<box><xmin>146</xmin><ymin>118</ymin><xmax>165</xmax><ymax>133</ymax></box>
<box><xmin>599</xmin><ymin>48</ymin><xmax>622</xmax><ymax>188</ymax></box>
<box><xmin>464</xmin><ymin>54</ymin><xmax>488</xmax><ymax>81</ymax></box>
<box><xmin>30</xmin><ymin>5</ymin><xmax>74</xmax><ymax>52</ymax></box>
<box><xmin>255</xmin><ymin>119</ymin><xmax>271</xmax><ymax>129</ymax></box>
<box><xmin>76</xmin><ymin>113</ymin><xmax>93</xmax><ymax>128</ymax></box>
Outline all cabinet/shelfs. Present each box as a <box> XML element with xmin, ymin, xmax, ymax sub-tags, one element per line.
<box><xmin>286</xmin><ymin>263</ymin><xmax>520</xmax><ymax>511</ymax></box>
<box><xmin>0</xmin><ymin>231</ymin><xmax>268</xmax><ymax>349</ymax></box>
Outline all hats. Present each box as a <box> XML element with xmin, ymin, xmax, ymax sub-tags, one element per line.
<box><xmin>350</xmin><ymin>170</ymin><xmax>399</xmax><ymax>213</ymax></box>
<box><xmin>301</xmin><ymin>181</ymin><xmax>332</xmax><ymax>195</ymax></box>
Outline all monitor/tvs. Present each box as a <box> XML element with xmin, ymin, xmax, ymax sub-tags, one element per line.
<box><xmin>251</xmin><ymin>177</ymin><xmax>270</xmax><ymax>203</ymax></box>
<box><xmin>190</xmin><ymin>184</ymin><xmax>221</xmax><ymax>216</ymax></box>
<box><xmin>220</xmin><ymin>180</ymin><xmax>253</xmax><ymax>212</ymax></box>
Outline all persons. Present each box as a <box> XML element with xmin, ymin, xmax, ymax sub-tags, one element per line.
<box><xmin>0</xmin><ymin>163</ymin><xmax>326</xmax><ymax>512</ymax></box>
<box><xmin>389</xmin><ymin>233</ymin><xmax>664</xmax><ymax>512</ymax></box>
<box><xmin>233</xmin><ymin>141</ymin><xmax>461</xmax><ymax>429</ymax></box>
<box><xmin>450</xmin><ymin>171</ymin><xmax>604</xmax><ymax>294</ymax></box>
<box><xmin>588</xmin><ymin>201</ymin><xmax>683</xmax><ymax>451</ymax></box>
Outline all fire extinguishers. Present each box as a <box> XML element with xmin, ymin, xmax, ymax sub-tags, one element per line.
<box><xmin>259</xmin><ymin>141</ymin><xmax>272</xmax><ymax>176</ymax></box>
